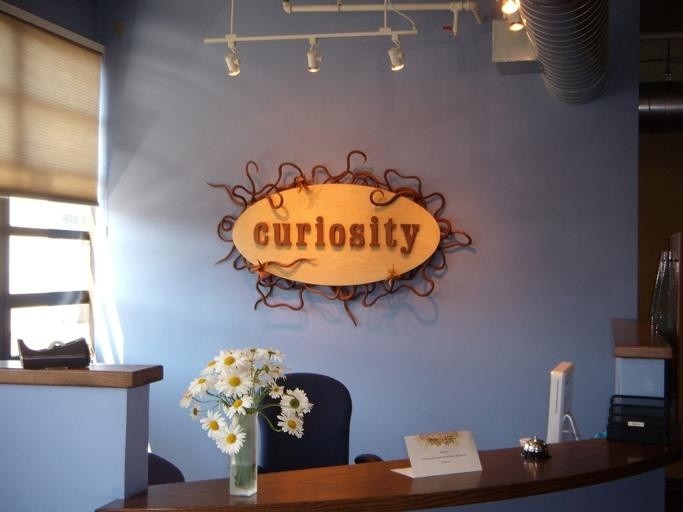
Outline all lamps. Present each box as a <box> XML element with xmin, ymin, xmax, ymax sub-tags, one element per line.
<box><xmin>201</xmin><ymin>30</ymin><xmax>419</xmax><ymax>77</ymax></box>
<box><xmin>500</xmin><ymin>0</ymin><xmax>524</xmax><ymax>32</ymax></box>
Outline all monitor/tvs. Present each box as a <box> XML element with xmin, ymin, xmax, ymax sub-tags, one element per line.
<box><xmin>546</xmin><ymin>362</ymin><xmax>580</xmax><ymax>444</ymax></box>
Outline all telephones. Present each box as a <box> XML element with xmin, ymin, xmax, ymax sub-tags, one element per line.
<box><xmin>17</xmin><ymin>337</ymin><xmax>89</xmax><ymax>370</ymax></box>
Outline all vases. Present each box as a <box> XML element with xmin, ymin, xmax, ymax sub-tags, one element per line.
<box><xmin>227</xmin><ymin>410</ymin><xmax>259</xmax><ymax>498</ymax></box>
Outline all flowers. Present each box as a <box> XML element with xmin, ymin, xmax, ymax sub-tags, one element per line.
<box><xmin>178</xmin><ymin>346</ymin><xmax>318</xmax><ymax>457</ymax></box>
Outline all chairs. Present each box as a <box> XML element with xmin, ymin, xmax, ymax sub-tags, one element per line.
<box><xmin>253</xmin><ymin>371</ymin><xmax>353</xmax><ymax>476</ymax></box>
